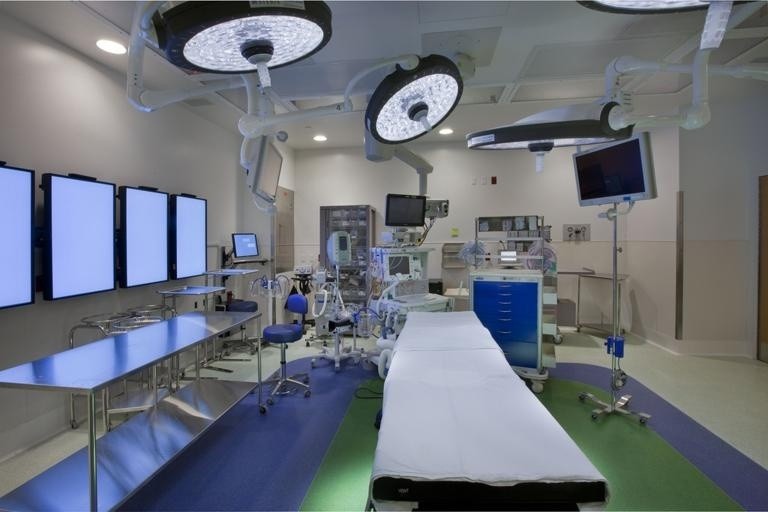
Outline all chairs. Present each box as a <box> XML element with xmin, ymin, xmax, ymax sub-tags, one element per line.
<box><xmin>263</xmin><ymin>294</ymin><xmax>313</xmax><ymax>405</ymax></box>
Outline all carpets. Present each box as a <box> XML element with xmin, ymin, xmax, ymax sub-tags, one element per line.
<box><xmin>111</xmin><ymin>355</ymin><xmax>768</xmax><ymax>511</ymax></box>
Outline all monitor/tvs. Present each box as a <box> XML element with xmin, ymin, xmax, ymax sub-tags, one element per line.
<box><xmin>571</xmin><ymin>131</ymin><xmax>658</xmax><ymax>207</ymax></box>
<box><xmin>384</xmin><ymin>193</ymin><xmax>427</xmax><ymax>227</ymax></box>
<box><xmin>252</xmin><ymin>136</ymin><xmax>283</xmax><ymax>204</ymax></box>
<box><xmin>232</xmin><ymin>232</ymin><xmax>259</xmax><ymax>258</ymax></box>
<box><xmin>383</xmin><ymin>254</ymin><xmax>413</xmax><ymax>279</ymax></box>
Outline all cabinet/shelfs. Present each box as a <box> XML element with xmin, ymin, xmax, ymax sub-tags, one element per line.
<box><xmin>240</xmin><ymin>186</ymin><xmax>295</xmax><ymax>337</ymax></box>
<box><xmin>318</xmin><ymin>204</ymin><xmax>376</xmax><ymax>337</ymax></box>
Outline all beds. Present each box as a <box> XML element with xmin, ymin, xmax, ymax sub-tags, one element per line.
<box><xmin>364</xmin><ymin>311</ymin><xmax>610</xmax><ymax>511</ymax></box>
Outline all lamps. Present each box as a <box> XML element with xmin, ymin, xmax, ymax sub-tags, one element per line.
<box><xmin>153</xmin><ymin>0</ymin><xmax>332</xmax><ymax>89</ymax></box>
<box><xmin>576</xmin><ymin>0</ymin><xmax>756</xmax><ymax>15</ymax></box>
<box><xmin>366</xmin><ymin>55</ymin><xmax>463</xmax><ymax>146</ymax></box>
<box><xmin>466</xmin><ymin>93</ymin><xmax>633</xmax><ymax>172</ymax></box>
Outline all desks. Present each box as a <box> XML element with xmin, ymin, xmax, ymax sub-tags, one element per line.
<box><xmin>0</xmin><ymin>310</ymin><xmax>265</xmax><ymax>512</ymax></box>
<box><xmin>158</xmin><ymin>287</ymin><xmax>227</xmax><ymax>381</ymax></box>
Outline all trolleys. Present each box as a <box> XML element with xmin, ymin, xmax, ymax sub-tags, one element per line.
<box><xmin>542</xmin><ymin>269</ymin><xmax>563</xmax><ymax>345</ymax></box>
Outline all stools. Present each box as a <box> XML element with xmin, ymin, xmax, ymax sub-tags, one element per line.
<box><xmin>224</xmin><ymin>301</ymin><xmax>258</xmax><ymax>355</ymax></box>
